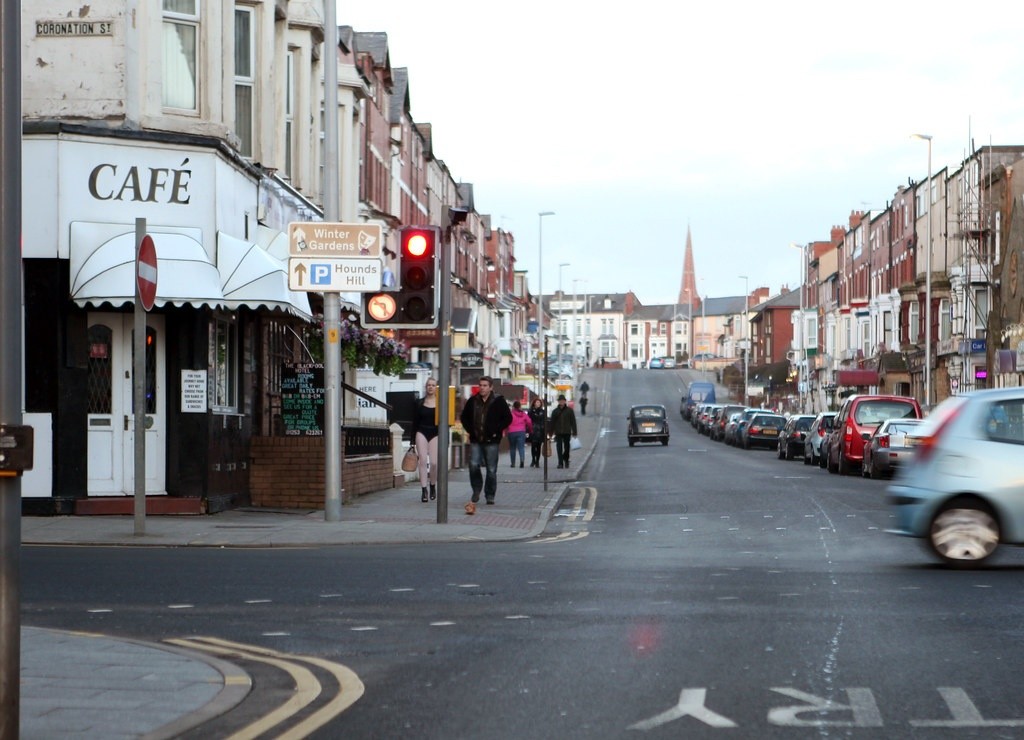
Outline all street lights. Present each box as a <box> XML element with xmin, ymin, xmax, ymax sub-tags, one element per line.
<box><xmin>911</xmin><ymin>133</ymin><xmax>930</xmax><ymax>407</ymax></box>
<box><xmin>791</xmin><ymin>244</ymin><xmax>802</xmax><ymax>407</ymax></box>
<box><xmin>684</xmin><ymin>288</ymin><xmax>690</xmax><ymax>359</ymax></box>
<box><xmin>559</xmin><ymin>263</ymin><xmax>570</xmax><ymax>380</ymax></box>
<box><xmin>738</xmin><ymin>276</ymin><xmax>748</xmax><ymax>395</ymax></box>
<box><xmin>538</xmin><ymin>211</ymin><xmax>556</xmax><ymax>399</ymax></box>
<box><xmin>590</xmin><ymin>296</ymin><xmax>595</xmax><ymax>320</ymax></box>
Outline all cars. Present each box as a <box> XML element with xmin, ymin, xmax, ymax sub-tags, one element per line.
<box><xmin>536</xmin><ymin>353</ymin><xmax>585</xmax><ymax>380</ymax></box>
<box><xmin>886</xmin><ymin>387</ymin><xmax>1024</xmax><ymax>568</ymax></box>
<box><xmin>826</xmin><ymin>394</ymin><xmax>923</xmax><ymax>476</ymax></box>
<box><xmin>627</xmin><ymin>404</ymin><xmax>670</xmax><ymax>447</ymax></box>
<box><xmin>862</xmin><ymin>418</ymin><xmax>926</xmax><ymax>480</ymax></box>
<box><xmin>776</xmin><ymin>415</ymin><xmax>816</xmax><ymax>461</ymax></box>
<box><xmin>650</xmin><ymin>353</ymin><xmax>718</xmax><ymax>370</ymax></box>
<box><xmin>804</xmin><ymin>412</ymin><xmax>838</xmax><ymax>467</ymax></box>
<box><xmin>690</xmin><ymin>404</ymin><xmax>787</xmax><ymax>450</ymax></box>
<box><xmin>819</xmin><ymin>413</ymin><xmax>840</xmax><ymax>469</ymax></box>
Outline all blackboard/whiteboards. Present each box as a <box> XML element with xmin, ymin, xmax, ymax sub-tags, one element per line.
<box><xmin>279</xmin><ymin>362</ymin><xmax>325</xmax><ymax>437</ymax></box>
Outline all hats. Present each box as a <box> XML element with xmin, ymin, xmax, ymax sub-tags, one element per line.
<box><xmin>558</xmin><ymin>394</ymin><xmax>568</xmax><ymax>402</ymax></box>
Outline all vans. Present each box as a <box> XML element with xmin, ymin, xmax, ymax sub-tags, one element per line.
<box><xmin>679</xmin><ymin>382</ymin><xmax>716</xmax><ymax>422</ymax></box>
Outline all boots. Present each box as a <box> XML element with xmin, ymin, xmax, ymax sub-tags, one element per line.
<box><xmin>429</xmin><ymin>483</ymin><xmax>436</xmax><ymax>499</ymax></box>
<box><xmin>422</xmin><ymin>486</ymin><xmax>428</xmax><ymax>501</ymax></box>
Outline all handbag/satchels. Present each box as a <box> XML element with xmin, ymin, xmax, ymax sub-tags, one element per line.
<box><xmin>401</xmin><ymin>446</ymin><xmax>418</xmax><ymax>472</ymax></box>
<box><xmin>543</xmin><ymin>437</ymin><xmax>551</xmax><ymax>456</ymax></box>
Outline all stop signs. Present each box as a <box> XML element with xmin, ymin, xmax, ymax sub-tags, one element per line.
<box><xmin>137</xmin><ymin>235</ymin><xmax>158</xmax><ymax>312</ymax></box>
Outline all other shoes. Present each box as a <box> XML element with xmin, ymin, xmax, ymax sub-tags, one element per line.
<box><xmin>472</xmin><ymin>488</ymin><xmax>482</xmax><ymax>503</ymax></box>
<box><xmin>564</xmin><ymin>461</ymin><xmax>569</xmax><ymax>467</ymax></box>
<box><xmin>557</xmin><ymin>464</ymin><xmax>564</xmax><ymax>469</ymax></box>
<box><xmin>487</xmin><ymin>494</ymin><xmax>495</xmax><ymax>505</ymax></box>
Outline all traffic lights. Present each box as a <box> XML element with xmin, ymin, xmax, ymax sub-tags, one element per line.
<box><xmin>361</xmin><ymin>224</ymin><xmax>439</xmax><ymax>329</ymax></box>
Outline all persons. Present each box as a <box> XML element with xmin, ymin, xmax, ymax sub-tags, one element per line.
<box><xmin>592</xmin><ymin>356</ymin><xmax>607</xmax><ymax>369</ymax></box>
<box><xmin>458</xmin><ymin>376</ymin><xmax>513</xmax><ymax>505</ymax></box>
<box><xmin>507</xmin><ymin>400</ymin><xmax>533</xmax><ymax>470</ymax></box>
<box><xmin>579</xmin><ymin>381</ymin><xmax>591</xmax><ymax>414</ymax></box>
<box><xmin>528</xmin><ymin>399</ymin><xmax>545</xmax><ymax>468</ymax></box>
<box><xmin>411</xmin><ymin>376</ymin><xmax>438</xmax><ymax>502</ymax></box>
<box><xmin>548</xmin><ymin>394</ymin><xmax>578</xmax><ymax>469</ymax></box>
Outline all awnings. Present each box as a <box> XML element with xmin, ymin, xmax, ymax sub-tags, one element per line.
<box><xmin>69</xmin><ymin>222</ymin><xmax>226</xmax><ymax>311</ymax></box>
<box><xmin>215</xmin><ymin>234</ymin><xmax>314</xmax><ymax>322</ymax></box>
<box><xmin>250</xmin><ymin>225</ymin><xmax>295</xmax><ymax>260</ymax></box>
